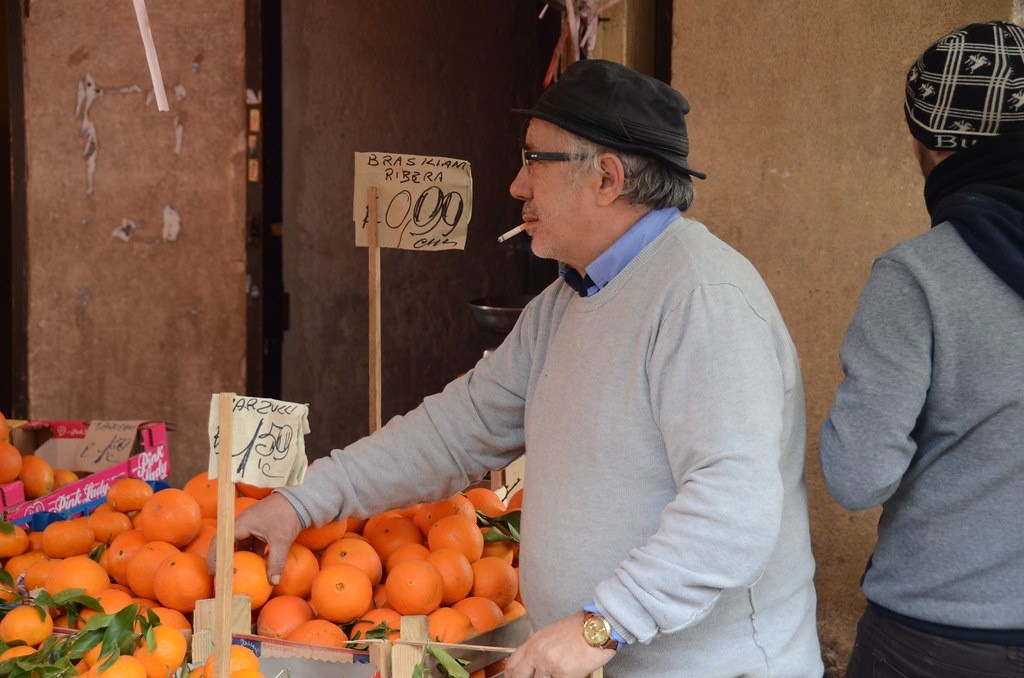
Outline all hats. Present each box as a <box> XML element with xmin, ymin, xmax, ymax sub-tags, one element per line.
<box><xmin>511</xmin><ymin>59</ymin><xmax>708</xmax><ymax>180</ymax></box>
<box><xmin>904</xmin><ymin>20</ymin><xmax>1024</xmax><ymax>150</ymax></box>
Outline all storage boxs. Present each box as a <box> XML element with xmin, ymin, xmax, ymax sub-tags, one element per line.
<box><xmin>192</xmin><ymin>593</ymin><xmax>533</xmax><ymax>678</ymax></box>
<box><xmin>0</xmin><ymin>419</ymin><xmax>172</xmax><ymax>533</ymax></box>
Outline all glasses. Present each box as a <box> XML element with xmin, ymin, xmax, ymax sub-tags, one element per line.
<box><xmin>522</xmin><ymin>147</ymin><xmax>599</xmax><ymax>174</ymax></box>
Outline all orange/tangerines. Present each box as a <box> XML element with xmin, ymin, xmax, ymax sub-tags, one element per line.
<box><xmin>230</xmin><ymin>487</ymin><xmax>528</xmax><ymax>678</ymax></box>
<box><xmin>0</xmin><ymin>410</ymin><xmax>220</xmax><ymax>678</ymax></box>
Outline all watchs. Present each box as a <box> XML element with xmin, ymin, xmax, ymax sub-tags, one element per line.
<box><xmin>583</xmin><ymin>611</ymin><xmax>617</xmax><ymax>650</ymax></box>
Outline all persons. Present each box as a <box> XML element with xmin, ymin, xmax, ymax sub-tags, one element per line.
<box><xmin>206</xmin><ymin>58</ymin><xmax>825</xmax><ymax>678</ymax></box>
<box><xmin>819</xmin><ymin>20</ymin><xmax>1024</xmax><ymax>677</ymax></box>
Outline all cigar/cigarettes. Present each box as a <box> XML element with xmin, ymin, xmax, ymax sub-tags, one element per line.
<box><xmin>498</xmin><ymin>223</ymin><xmax>527</xmax><ymax>243</ymax></box>
